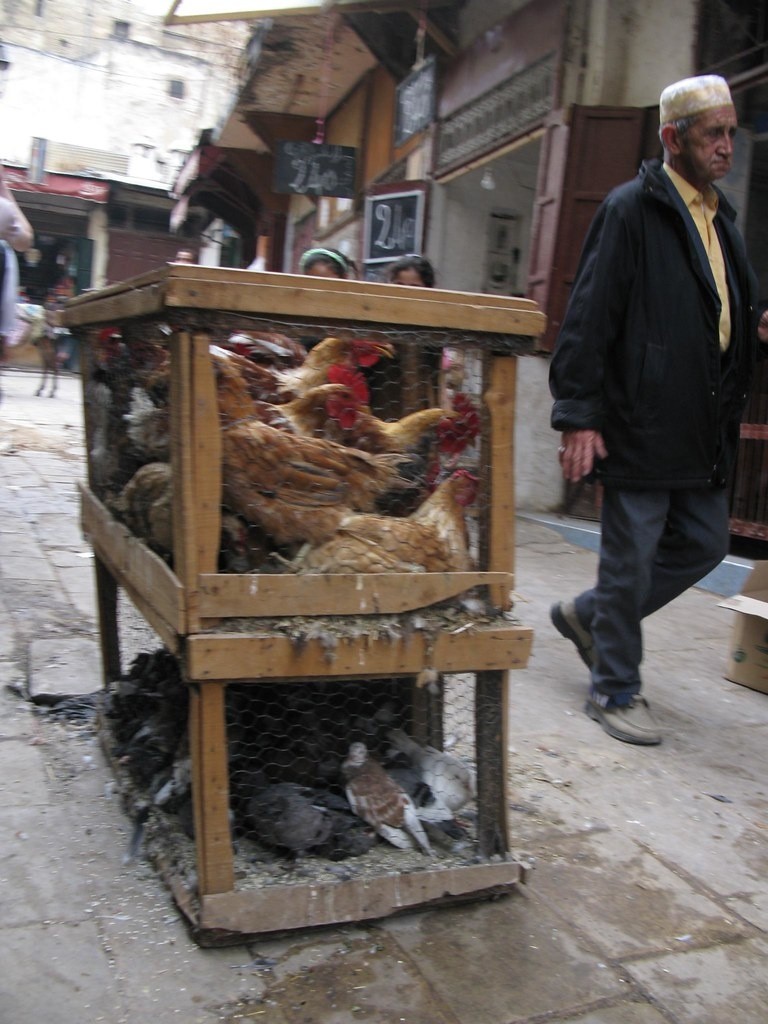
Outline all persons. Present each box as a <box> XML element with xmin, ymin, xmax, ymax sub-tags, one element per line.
<box><xmin>299</xmin><ymin>246</ymin><xmax>357</xmax><ymax>278</ymax></box>
<box><xmin>384</xmin><ymin>255</ymin><xmax>436</xmax><ymax>288</ymax></box>
<box><xmin>173</xmin><ymin>248</ymin><xmax>198</xmax><ymax>264</ymax></box>
<box><xmin>0</xmin><ymin>179</ymin><xmax>35</xmax><ymax>406</ymax></box>
<box><xmin>548</xmin><ymin>76</ymin><xmax>768</xmax><ymax>746</ymax></box>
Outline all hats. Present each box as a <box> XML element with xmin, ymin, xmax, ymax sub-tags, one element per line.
<box><xmin>658</xmin><ymin>74</ymin><xmax>734</xmax><ymax>124</ymax></box>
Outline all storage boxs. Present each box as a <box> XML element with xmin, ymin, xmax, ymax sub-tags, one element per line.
<box><xmin>716</xmin><ymin>560</ymin><xmax>768</xmax><ymax>697</ymax></box>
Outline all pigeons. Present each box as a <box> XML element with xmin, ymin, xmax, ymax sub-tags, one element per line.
<box><xmin>112</xmin><ymin>645</ymin><xmax>478</xmax><ymax>858</ymax></box>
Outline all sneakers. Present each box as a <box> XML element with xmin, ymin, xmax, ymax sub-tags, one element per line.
<box><xmin>550</xmin><ymin>598</ymin><xmax>595</xmax><ymax>671</ymax></box>
<box><xmin>582</xmin><ymin>691</ymin><xmax>663</xmax><ymax>745</ymax></box>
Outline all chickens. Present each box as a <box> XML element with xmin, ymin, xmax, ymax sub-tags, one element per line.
<box><xmin>93</xmin><ymin>321</ymin><xmax>481</xmax><ymax>574</ymax></box>
